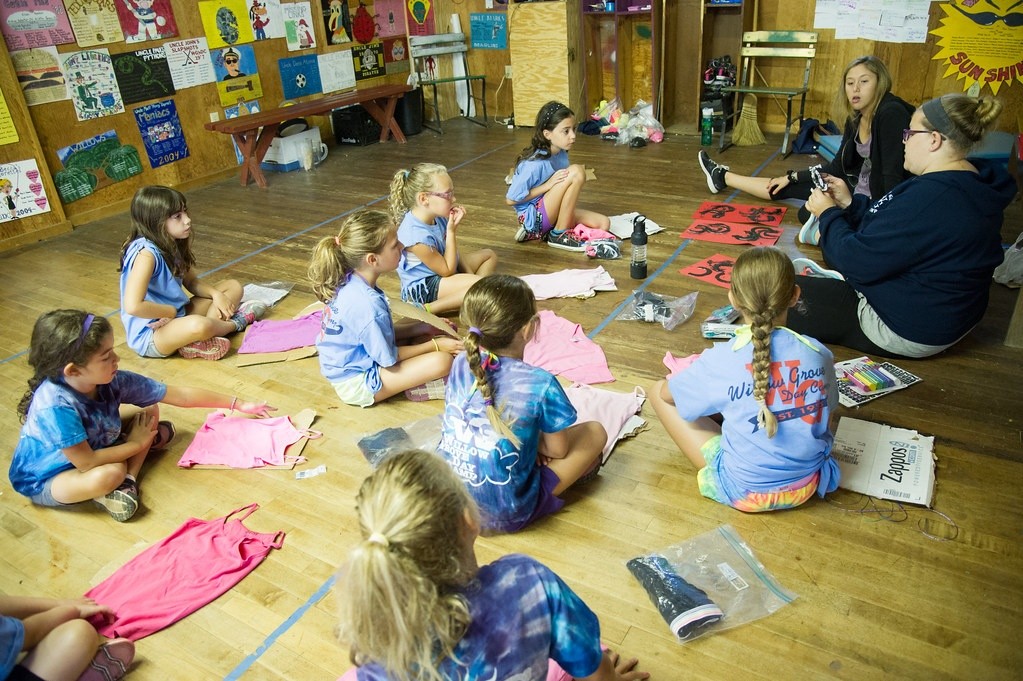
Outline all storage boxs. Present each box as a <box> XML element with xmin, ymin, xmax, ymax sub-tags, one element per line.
<box><xmin>232</xmin><ymin>123</ymin><xmax>323</xmax><ymax>172</ymax></box>
<box><xmin>332</xmin><ymin>105</ymin><xmax>386</xmax><ymax>146</ymax></box>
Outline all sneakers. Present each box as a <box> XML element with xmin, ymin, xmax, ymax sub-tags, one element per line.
<box><xmin>229</xmin><ymin>300</ymin><xmax>267</xmax><ymax>331</ymax></box>
<box><xmin>704</xmin><ymin>67</ymin><xmax>732</xmax><ymax>85</ymax></box>
<box><xmin>150</xmin><ymin>420</ymin><xmax>176</xmax><ymax>449</ymax></box>
<box><xmin>799</xmin><ymin>212</ymin><xmax>822</xmax><ymax>244</ymax></box>
<box><xmin>698</xmin><ymin>149</ymin><xmax>726</xmax><ymax>194</ymax></box>
<box><xmin>791</xmin><ymin>257</ymin><xmax>844</xmax><ymax>282</ymax></box>
<box><xmin>548</xmin><ymin>229</ymin><xmax>589</xmax><ymax>252</ymax></box>
<box><xmin>515</xmin><ymin>228</ymin><xmax>543</xmax><ymax>242</ymax></box>
<box><xmin>178</xmin><ymin>336</ymin><xmax>231</xmax><ymax>361</ymax></box>
<box><xmin>91</xmin><ymin>478</ymin><xmax>139</xmax><ymax>522</ymax></box>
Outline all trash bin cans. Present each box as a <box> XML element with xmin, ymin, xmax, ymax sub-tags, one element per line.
<box><xmin>375</xmin><ymin>87</ymin><xmax>424</xmax><ymax>135</ymax></box>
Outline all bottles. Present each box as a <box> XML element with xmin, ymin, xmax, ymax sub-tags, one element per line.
<box><xmin>630</xmin><ymin>215</ymin><xmax>648</xmax><ymax>279</ymax></box>
<box><xmin>701</xmin><ymin>109</ymin><xmax>712</xmax><ymax>146</ymax></box>
<box><xmin>606</xmin><ymin>1</ymin><xmax>614</xmax><ymax>12</ymax></box>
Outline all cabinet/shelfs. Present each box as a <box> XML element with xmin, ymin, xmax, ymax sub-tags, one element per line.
<box><xmin>580</xmin><ymin>0</ymin><xmax>755</xmax><ymax>140</ymax></box>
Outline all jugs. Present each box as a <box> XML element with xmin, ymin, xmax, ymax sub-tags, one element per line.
<box><xmin>294</xmin><ymin>137</ymin><xmax>329</xmax><ymax>171</ymax></box>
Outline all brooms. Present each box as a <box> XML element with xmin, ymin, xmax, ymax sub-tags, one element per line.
<box><xmin>732</xmin><ymin>0</ymin><xmax>769</xmax><ymax>147</ymax></box>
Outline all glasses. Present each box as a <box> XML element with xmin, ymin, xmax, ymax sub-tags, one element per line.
<box><xmin>902</xmin><ymin>129</ymin><xmax>948</xmax><ymax>142</ymax></box>
<box><xmin>426</xmin><ymin>191</ymin><xmax>457</xmax><ymax>200</ymax></box>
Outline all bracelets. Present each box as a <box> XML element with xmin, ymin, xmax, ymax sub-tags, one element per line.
<box><xmin>787</xmin><ymin>170</ymin><xmax>797</xmax><ymax>183</ymax></box>
<box><xmin>231</xmin><ymin>396</ymin><xmax>237</xmax><ymax>411</ymax></box>
<box><xmin>432</xmin><ymin>339</ymin><xmax>439</xmax><ymax>351</ymax></box>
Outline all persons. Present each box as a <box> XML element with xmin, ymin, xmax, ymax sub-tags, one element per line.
<box><xmin>697</xmin><ymin>56</ymin><xmax>916</xmax><ymax>225</ymax></box>
<box><xmin>387</xmin><ymin>163</ymin><xmax>498</xmax><ymax>314</ymax></box>
<box><xmin>115</xmin><ymin>185</ymin><xmax>267</xmax><ymax>361</ymax></box>
<box><xmin>506</xmin><ymin>101</ymin><xmax>610</xmax><ymax>251</ymax></box>
<box><xmin>646</xmin><ymin>246</ymin><xmax>841</xmax><ymax>511</ymax></box>
<box><xmin>434</xmin><ymin>275</ymin><xmax>608</xmax><ymax>535</ymax></box>
<box><xmin>337</xmin><ymin>448</ymin><xmax>650</xmax><ymax>681</ymax></box>
<box><xmin>8</xmin><ymin>309</ymin><xmax>278</xmax><ymax>522</ymax></box>
<box><xmin>0</xmin><ymin>588</ymin><xmax>135</xmax><ymax>681</ymax></box>
<box><xmin>307</xmin><ymin>211</ymin><xmax>464</xmax><ymax>410</ymax></box>
<box><xmin>787</xmin><ymin>94</ymin><xmax>1019</xmax><ymax>359</ymax></box>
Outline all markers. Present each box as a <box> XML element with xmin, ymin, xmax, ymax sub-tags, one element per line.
<box><xmin>842</xmin><ymin>363</ymin><xmax>895</xmax><ymax>391</ymax></box>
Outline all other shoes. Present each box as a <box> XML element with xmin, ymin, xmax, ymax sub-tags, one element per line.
<box><xmin>404</xmin><ymin>376</ymin><xmax>448</xmax><ymax>401</ymax></box>
<box><xmin>78</xmin><ymin>637</ymin><xmax>135</xmax><ymax>681</ymax></box>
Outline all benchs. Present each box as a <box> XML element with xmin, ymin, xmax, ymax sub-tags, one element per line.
<box><xmin>203</xmin><ymin>83</ymin><xmax>413</xmax><ymax>187</ymax></box>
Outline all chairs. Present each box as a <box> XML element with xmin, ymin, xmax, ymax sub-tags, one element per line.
<box><xmin>718</xmin><ymin>29</ymin><xmax>818</xmax><ymax>160</ymax></box>
<box><xmin>409</xmin><ymin>34</ymin><xmax>487</xmax><ymax>136</ymax></box>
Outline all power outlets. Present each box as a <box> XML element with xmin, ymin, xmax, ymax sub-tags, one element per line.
<box><xmin>504</xmin><ymin>65</ymin><xmax>513</xmax><ymax>79</ymax></box>
